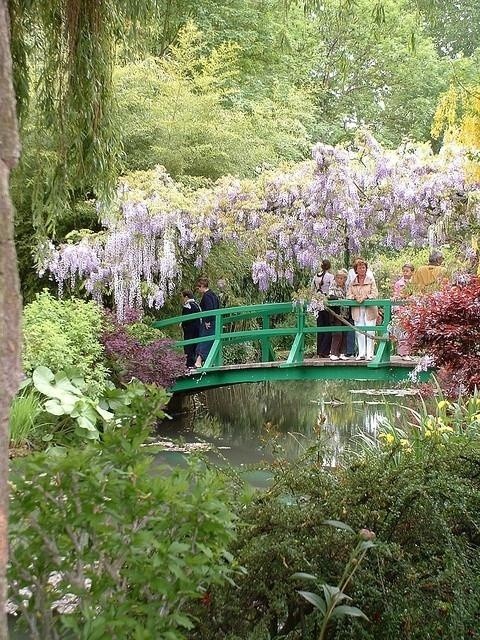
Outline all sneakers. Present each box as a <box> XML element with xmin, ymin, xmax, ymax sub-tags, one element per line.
<box><xmin>318</xmin><ymin>353</ymin><xmax>416</xmax><ymax>361</ymax></box>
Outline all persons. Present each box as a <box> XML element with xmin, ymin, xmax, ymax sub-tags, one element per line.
<box><xmin>344</xmin><ymin>258</ymin><xmax>375</xmax><ymax>358</ymax></box>
<box><xmin>402</xmin><ymin>251</ymin><xmax>447</xmax><ymax>301</ymax></box>
<box><xmin>394</xmin><ymin>264</ymin><xmax>414</xmax><ymax>360</ymax></box>
<box><xmin>179</xmin><ymin>288</ymin><xmax>200</xmax><ymax>368</ymax></box>
<box><xmin>189</xmin><ymin>278</ymin><xmax>219</xmax><ymax>368</ymax></box>
<box><xmin>329</xmin><ymin>269</ymin><xmax>348</xmax><ymax>360</ymax></box>
<box><xmin>311</xmin><ymin>260</ymin><xmax>336</xmax><ymax>358</ymax></box>
<box><xmin>347</xmin><ymin>260</ymin><xmax>379</xmax><ymax>360</ymax></box>
